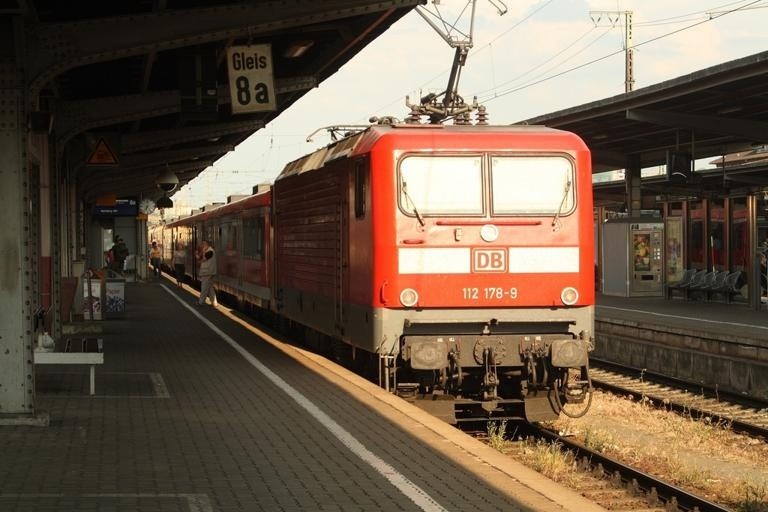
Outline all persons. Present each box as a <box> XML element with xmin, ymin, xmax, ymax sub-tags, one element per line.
<box><xmin>193</xmin><ymin>240</ymin><xmax>219</xmax><ymax>307</ymax></box>
<box><xmin>150</xmin><ymin>241</ymin><xmax>164</xmax><ymax>279</ymax></box>
<box><xmin>117</xmin><ymin>238</ymin><xmax>127</xmax><ymax>258</ymax></box>
<box><xmin>170</xmin><ymin>240</ymin><xmax>186</xmax><ymax>289</ymax></box>
<box><xmin>112</xmin><ymin>235</ymin><xmax>127</xmax><ymax>273</ymax></box>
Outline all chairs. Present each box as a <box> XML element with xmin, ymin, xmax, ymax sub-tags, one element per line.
<box><xmin>663</xmin><ymin>266</ymin><xmax>742</xmax><ymax>306</ymax></box>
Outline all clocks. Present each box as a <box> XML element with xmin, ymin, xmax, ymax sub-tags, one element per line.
<box><xmin>138</xmin><ymin>198</ymin><xmax>156</xmax><ymax>215</ymax></box>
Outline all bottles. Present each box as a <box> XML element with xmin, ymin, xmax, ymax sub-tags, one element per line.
<box><xmin>38</xmin><ymin>331</ymin><xmax>55</xmax><ymax>349</ymax></box>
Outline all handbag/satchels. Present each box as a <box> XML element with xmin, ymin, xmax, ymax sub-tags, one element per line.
<box><xmin>150</xmin><ymin>257</ymin><xmax>160</xmax><ymax>268</ymax></box>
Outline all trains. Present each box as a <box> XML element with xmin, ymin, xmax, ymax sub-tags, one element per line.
<box><xmin>146</xmin><ymin>95</ymin><xmax>594</xmax><ymax>443</ymax></box>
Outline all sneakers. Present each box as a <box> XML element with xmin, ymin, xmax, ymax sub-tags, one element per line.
<box><xmin>154</xmin><ymin>273</ymin><xmax>219</xmax><ymax>311</ymax></box>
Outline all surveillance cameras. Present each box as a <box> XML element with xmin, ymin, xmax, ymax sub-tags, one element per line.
<box><xmin>155</xmin><ymin>167</ymin><xmax>179</xmax><ymax>193</ymax></box>
